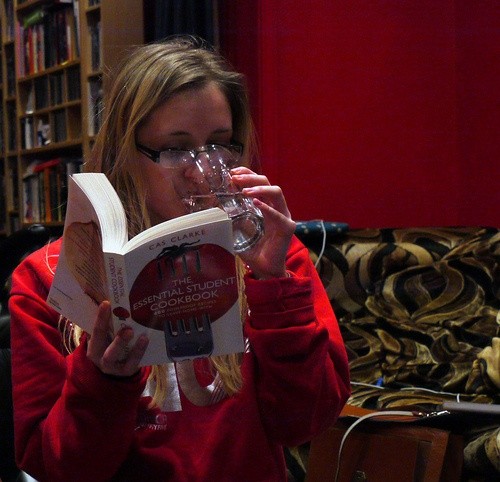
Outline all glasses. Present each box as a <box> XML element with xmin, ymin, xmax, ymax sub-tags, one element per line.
<box><xmin>133</xmin><ymin>141</ymin><xmax>242</xmax><ymax>168</ymax></box>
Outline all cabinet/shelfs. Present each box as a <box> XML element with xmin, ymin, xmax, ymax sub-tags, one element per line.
<box><xmin>0</xmin><ymin>0</ymin><xmax>143</xmax><ymax>229</ymax></box>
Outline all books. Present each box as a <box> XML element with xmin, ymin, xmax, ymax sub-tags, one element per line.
<box><xmin>45</xmin><ymin>174</ymin><xmax>249</xmax><ymax>370</ymax></box>
<box><xmin>0</xmin><ymin>2</ymin><xmax>102</xmax><ymax>230</ymax></box>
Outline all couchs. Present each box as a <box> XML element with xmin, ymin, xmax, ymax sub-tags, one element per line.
<box><xmin>0</xmin><ymin>221</ymin><xmax>500</xmax><ymax>482</ymax></box>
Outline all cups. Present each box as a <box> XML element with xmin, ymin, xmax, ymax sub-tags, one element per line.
<box><xmin>170</xmin><ymin>145</ymin><xmax>265</xmax><ymax>252</ymax></box>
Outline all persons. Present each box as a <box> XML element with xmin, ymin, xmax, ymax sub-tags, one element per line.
<box><xmin>6</xmin><ymin>38</ymin><xmax>353</xmax><ymax>482</ymax></box>
